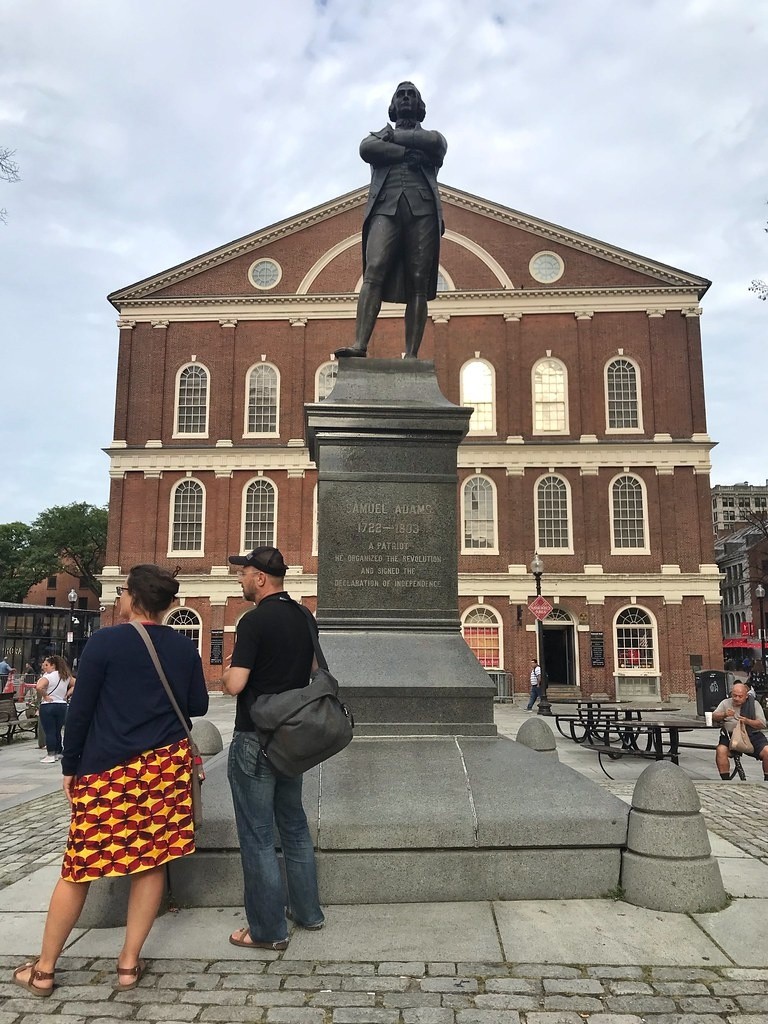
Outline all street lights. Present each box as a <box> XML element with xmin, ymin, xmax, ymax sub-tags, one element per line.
<box><xmin>755</xmin><ymin>583</ymin><xmax>766</xmax><ymax>678</ymax></box>
<box><xmin>65</xmin><ymin>588</ymin><xmax>77</xmax><ymax>674</ymax></box>
<box><xmin>530</xmin><ymin>551</ymin><xmax>553</xmax><ymax>717</ymax></box>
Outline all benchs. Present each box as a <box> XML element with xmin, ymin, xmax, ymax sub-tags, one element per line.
<box><xmin>0</xmin><ymin>692</ymin><xmax>39</xmax><ymax>744</ymax></box>
<box><xmin>543</xmin><ymin>712</ymin><xmax>741</xmax><ymax>757</ymax></box>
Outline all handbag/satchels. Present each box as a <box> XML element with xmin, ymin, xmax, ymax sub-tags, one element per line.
<box><xmin>191</xmin><ymin>744</ymin><xmax>206</xmax><ymax>831</ymax></box>
<box><xmin>249</xmin><ymin>668</ymin><xmax>354</xmax><ymax>778</ymax></box>
<box><xmin>539</xmin><ymin>682</ymin><xmax>548</xmax><ymax>689</ymax></box>
<box><xmin>728</xmin><ymin>719</ymin><xmax>754</xmax><ymax>754</ymax></box>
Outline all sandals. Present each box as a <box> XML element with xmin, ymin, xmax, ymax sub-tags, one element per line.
<box><xmin>229</xmin><ymin>927</ymin><xmax>290</xmax><ymax>950</ymax></box>
<box><xmin>13</xmin><ymin>958</ymin><xmax>55</xmax><ymax>996</ymax></box>
<box><xmin>286</xmin><ymin>905</ymin><xmax>325</xmax><ymax>931</ymax></box>
<box><xmin>117</xmin><ymin>958</ymin><xmax>146</xmax><ymax>991</ymax></box>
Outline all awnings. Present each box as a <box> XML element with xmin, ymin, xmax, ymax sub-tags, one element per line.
<box><xmin>723</xmin><ymin>639</ymin><xmax>768</xmax><ymax>648</ymax></box>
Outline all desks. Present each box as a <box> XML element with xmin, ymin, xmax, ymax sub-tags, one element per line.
<box><xmin>611</xmin><ymin>721</ymin><xmax>722</xmax><ymax>766</ymax></box>
<box><xmin>577</xmin><ymin>707</ymin><xmax>680</xmax><ymax>745</ymax></box>
<box><xmin>547</xmin><ymin>697</ymin><xmax>632</xmax><ymax>739</ymax></box>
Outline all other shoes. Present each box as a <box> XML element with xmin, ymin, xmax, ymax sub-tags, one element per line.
<box><xmin>524</xmin><ymin>708</ymin><xmax>532</xmax><ymax>712</ymax></box>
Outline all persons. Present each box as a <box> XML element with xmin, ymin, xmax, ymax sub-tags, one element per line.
<box><xmin>36</xmin><ymin>655</ymin><xmax>76</xmax><ymax>763</ymax></box>
<box><xmin>0</xmin><ymin>657</ymin><xmax>12</xmax><ymax>692</ymax></box>
<box><xmin>12</xmin><ymin>564</ymin><xmax>209</xmax><ymax>997</ymax></box>
<box><xmin>220</xmin><ymin>546</ymin><xmax>324</xmax><ymax>949</ymax></box>
<box><xmin>524</xmin><ymin>659</ymin><xmax>541</xmax><ymax>710</ymax></box>
<box><xmin>743</xmin><ymin>656</ymin><xmax>750</xmax><ymax>666</ymax></box>
<box><xmin>25</xmin><ymin>662</ymin><xmax>35</xmax><ymax>693</ymax></box>
<box><xmin>712</xmin><ymin>684</ymin><xmax>768</xmax><ymax>781</ymax></box>
<box><xmin>334</xmin><ymin>81</ymin><xmax>448</xmax><ymax>357</ymax></box>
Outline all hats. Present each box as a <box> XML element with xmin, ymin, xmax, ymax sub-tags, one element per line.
<box><xmin>229</xmin><ymin>546</ymin><xmax>286</xmax><ymax>576</ymax></box>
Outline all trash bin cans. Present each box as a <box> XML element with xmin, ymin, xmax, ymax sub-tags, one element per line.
<box><xmin>695</xmin><ymin>669</ymin><xmax>726</xmax><ymax>715</ymax></box>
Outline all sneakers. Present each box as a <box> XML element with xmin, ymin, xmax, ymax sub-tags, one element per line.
<box><xmin>40</xmin><ymin>755</ymin><xmax>58</xmax><ymax>763</ymax></box>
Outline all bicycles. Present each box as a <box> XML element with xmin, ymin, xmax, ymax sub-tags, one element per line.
<box><xmin>16</xmin><ymin>685</ymin><xmax>39</xmax><ymax>731</ymax></box>
